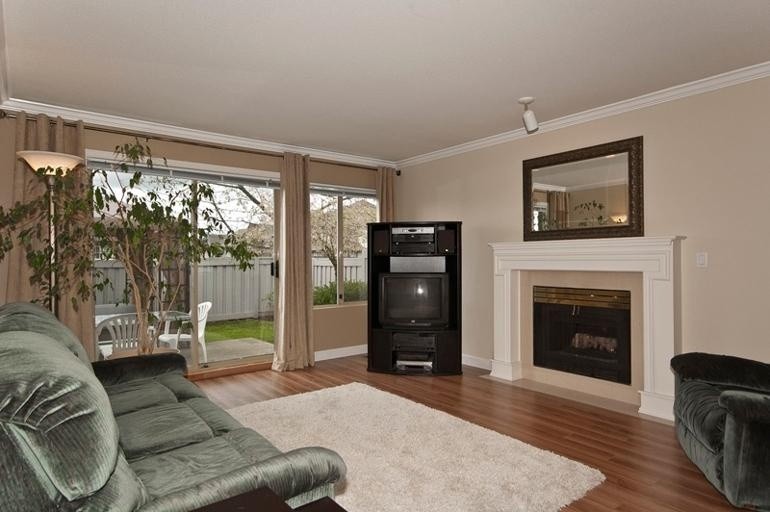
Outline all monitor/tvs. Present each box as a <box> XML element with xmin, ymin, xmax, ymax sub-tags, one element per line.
<box><xmin>380</xmin><ymin>273</ymin><xmax>449</xmax><ymax>325</ymax></box>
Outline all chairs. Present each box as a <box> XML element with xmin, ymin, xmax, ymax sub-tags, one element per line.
<box><xmin>669</xmin><ymin>352</ymin><xmax>770</xmax><ymax>512</ymax></box>
<box><xmin>96</xmin><ymin>312</ymin><xmax>157</xmax><ymax>360</ymax></box>
<box><xmin>158</xmin><ymin>301</ymin><xmax>213</xmax><ymax>368</ymax></box>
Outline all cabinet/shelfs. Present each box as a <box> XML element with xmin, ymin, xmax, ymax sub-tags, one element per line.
<box><xmin>366</xmin><ymin>221</ymin><xmax>463</xmax><ymax>376</ymax></box>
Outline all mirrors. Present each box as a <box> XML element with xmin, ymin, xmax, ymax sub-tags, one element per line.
<box><xmin>522</xmin><ymin>135</ymin><xmax>644</xmax><ymax>242</ymax></box>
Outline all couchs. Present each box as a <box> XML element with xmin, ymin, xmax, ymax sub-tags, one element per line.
<box><xmin>0</xmin><ymin>301</ymin><xmax>347</xmax><ymax>512</ymax></box>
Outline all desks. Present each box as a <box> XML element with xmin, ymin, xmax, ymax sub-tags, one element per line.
<box><xmin>94</xmin><ymin>311</ymin><xmax>191</xmax><ymax>353</ymax></box>
<box><xmin>193</xmin><ymin>486</ymin><xmax>347</xmax><ymax>512</ymax></box>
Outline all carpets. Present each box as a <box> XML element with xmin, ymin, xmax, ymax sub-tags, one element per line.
<box><xmin>224</xmin><ymin>380</ymin><xmax>607</xmax><ymax>512</ymax></box>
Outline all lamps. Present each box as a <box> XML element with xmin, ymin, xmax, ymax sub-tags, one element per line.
<box><xmin>517</xmin><ymin>97</ymin><xmax>539</xmax><ymax>134</ymax></box>
<box><xmin>16</xmin><ymin>149</ymin><xmax>85</xmax><ymax>318</ymax></box>
